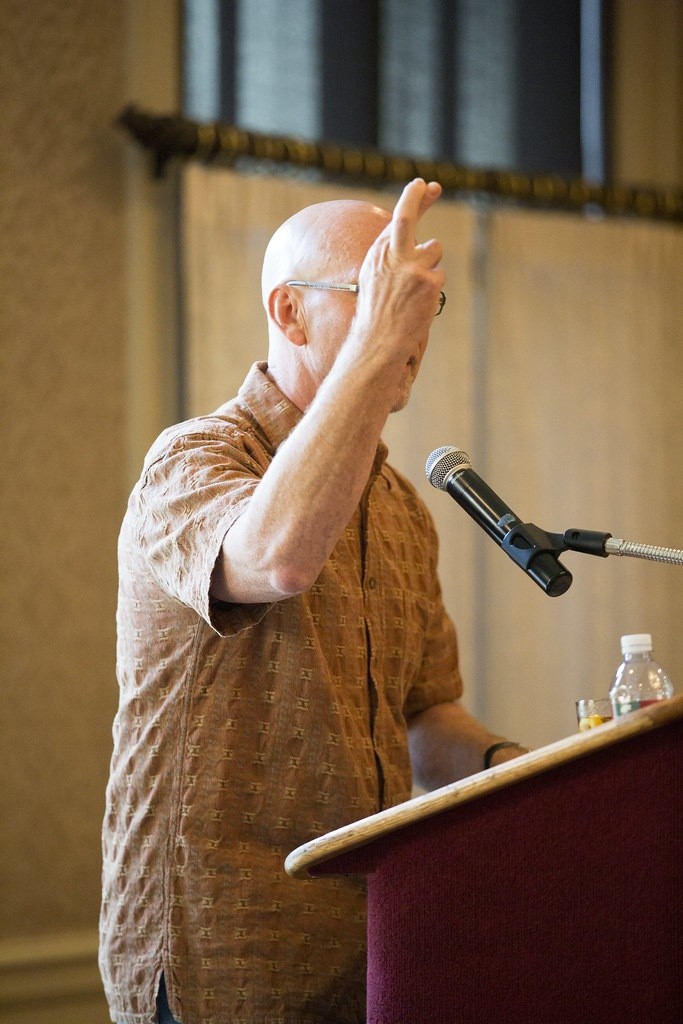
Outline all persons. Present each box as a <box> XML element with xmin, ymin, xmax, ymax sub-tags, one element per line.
<box><xmin>95</xmin><ymin>178</ymin><xmax>527</xmax><ymax>1024</ymax></box>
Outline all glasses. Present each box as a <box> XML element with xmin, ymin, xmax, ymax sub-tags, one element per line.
<box><xmin>285</xmin><ymin>280</ymin><xmax>446</xmax><ymax>316</ymax></box>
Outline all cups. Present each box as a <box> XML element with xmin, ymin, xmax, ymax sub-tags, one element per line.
<box><xmin>576</xmin><ymin>699</ymin><xmax>613</xmax><ymax>733</ymax></box>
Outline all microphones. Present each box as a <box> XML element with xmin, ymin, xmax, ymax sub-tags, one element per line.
<box><xmin>426</xmin><ymin>445</ymin><xmax>573</xmax><ymax>597</ymax></box>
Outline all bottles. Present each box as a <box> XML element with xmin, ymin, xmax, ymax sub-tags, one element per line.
<box><xmin>608</xmin><ymin>633</ymin><xmax>673</xmax><ymax>720</ymax></box>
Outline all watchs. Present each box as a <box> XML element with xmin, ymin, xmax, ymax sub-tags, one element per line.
<box><xmin>483</xmin><ymin>742</ymin><xmax>531</xmax><ymax>770</ymax></box>
